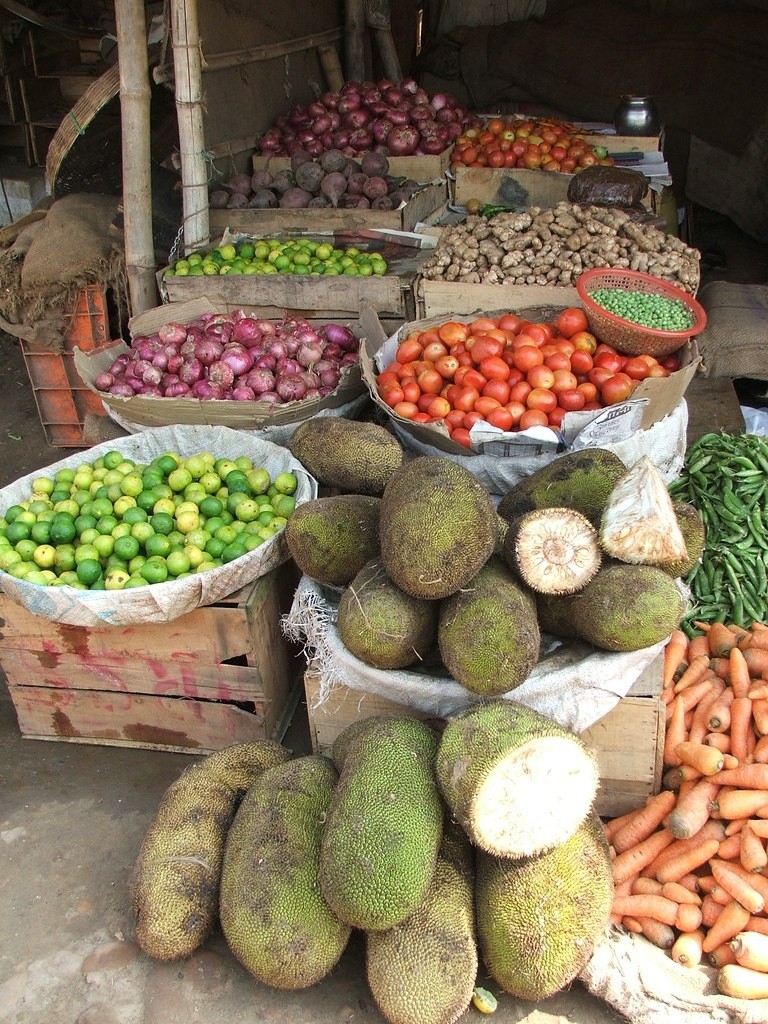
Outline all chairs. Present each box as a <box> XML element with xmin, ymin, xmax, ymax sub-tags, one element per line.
<box><xmin>691</xmin><ymin>280</ymin><xmax>768</xmax><ymax>411</ymax></box>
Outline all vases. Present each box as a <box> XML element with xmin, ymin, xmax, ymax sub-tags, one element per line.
<box><xmin>614</xmin><ymin>92</ymin><xmax>662</xmax><ymax>138</ymax></box>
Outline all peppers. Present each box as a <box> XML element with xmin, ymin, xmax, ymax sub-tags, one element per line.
<box><xmin>459</xmin><ymin>204</ymin><xmax>516</xmax><ymax>224</ymax></box>
<box><xmin>530</xmin><ymin>117</ymin><xmax>582</xmax><ymax>135</ymax></box>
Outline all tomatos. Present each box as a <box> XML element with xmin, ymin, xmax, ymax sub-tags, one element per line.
<box><xmin>376</xmin><ymin>309</ymin><xmax>680</xmax><ymax>449</ymax></box>
<box><xmin>462</xmin><ymin>119</ymin><xmax>615</xmax><ymax>176</ymax></box>
<box><xmin>466</xmin><ymin>199</ymin><xmax>480</xmax><ymax>214</ymax></box>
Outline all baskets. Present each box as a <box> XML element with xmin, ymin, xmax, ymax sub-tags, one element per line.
<box><xmin>577</xmin><ymin>268</ymin><xmax>708</xmax><ymax>356</ymax></box>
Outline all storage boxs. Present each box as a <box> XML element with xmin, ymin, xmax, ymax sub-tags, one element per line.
<box><xmin>0</xmin><ymin>105</ymin><xmax>703</xmax><ymax>817</ymax></box>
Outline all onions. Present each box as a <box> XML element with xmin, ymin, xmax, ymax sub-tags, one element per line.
<box><xmin>257</xmin><ymin>78</ymin><xmax>484</xmax><ymax>157</ymax></box>
<box><xmin>95</xmin><ymin>308</ymin><xmax>359</xmax><ymax>407</ymax></box>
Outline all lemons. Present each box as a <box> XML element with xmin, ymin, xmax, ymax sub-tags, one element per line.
<box><xmin>162</xmin><ymin>238</ymin><xmax>386</xmax><ymax>280</ymax></box>
<box><xmin>0</xmin><ymin>451</ymin><xmax>296</xmax><ymax>590</ymax></box>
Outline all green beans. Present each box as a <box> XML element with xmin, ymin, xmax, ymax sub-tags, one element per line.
<box><xmin>664</xmin><ymin>428</ymin><xmax>768</xmax><ymax>637</ymax></box>
<box><xmin>584</xmin><ymin>288</ymin><xmax>693</xmax><ymax>356</ymax></box>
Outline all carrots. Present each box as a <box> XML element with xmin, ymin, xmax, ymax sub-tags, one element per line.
<box><xmin>601</xmin><ymin>619</ymin><xmax>768</xmax><ymax>1001</ymax></box>
